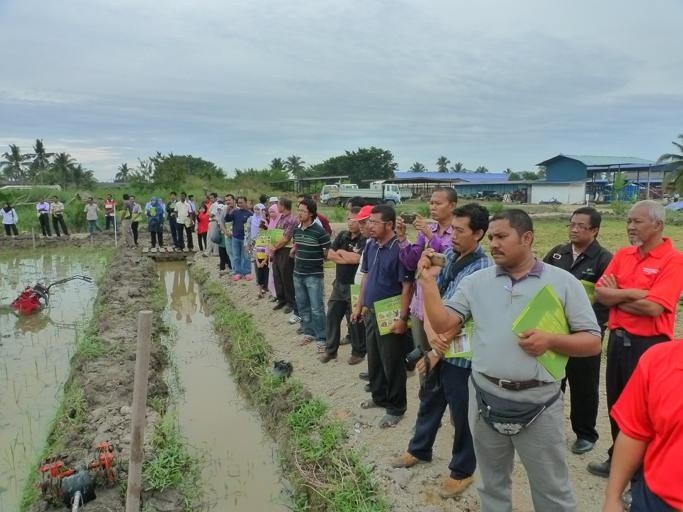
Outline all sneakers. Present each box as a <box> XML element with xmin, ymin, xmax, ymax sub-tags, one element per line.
<box><xmin>440</xmin><ymin>477</ymin><xmax>474</xmax><ymax>498</ymax></box>
<box><xmin>322</xmin><ymin>352</ymin><xmax>336</xmax><ymax>362</ymax></box>
<box><xmin>587</xmin><ymin>460</ymin><xmax>609</xmax><ymax>477</ymax></box>
<box><xmin>361</xmin><ymin>399</ymin><xmax>377</xmax><ymax>408</ymax></box>
<box><xmin>348</xmin><ymin>355</ymin><xmax>363</xmax><ymax>365</ymax></box>
<box><xmin>391</xmin><ymin>453</ymin><xmax>426</xmax><ymax>467</ymax></box>
<box><xmin>379</xmin><ymin>414</ymin><xmax>403</xmax><ymax>428</ymax></box>
<box><xmin>358</xmin><ymin>371</ymin><xmax>369</xmax><ymax>380</ymax></box>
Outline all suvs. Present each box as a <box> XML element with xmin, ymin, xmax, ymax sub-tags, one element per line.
<box><xmin>475</xmin><ymin>190</ymin><xmax>501</xmax><ymax>201</ymax></box>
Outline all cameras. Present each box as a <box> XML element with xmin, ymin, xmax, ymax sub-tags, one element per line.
<box><xmin>405</xmin><ymin>345</ymin><xmax>439</xmax><ymax>392</ymax></box>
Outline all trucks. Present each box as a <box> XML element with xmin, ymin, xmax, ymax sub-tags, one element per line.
<box><xmin>318</xmin><ymin>180</ymin><xmax>413</xmax><ymax>209</ymax></box>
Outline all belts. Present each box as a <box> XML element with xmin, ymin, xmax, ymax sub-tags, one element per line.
<box><xmin>481</xmin><ymin>372</ymin><xmax>550</xmax><ymax>390</ymax></box>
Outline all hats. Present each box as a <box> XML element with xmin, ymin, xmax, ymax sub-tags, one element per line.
<box><xmin>268</xmin><ymin>196</ymin><xmax>277</xmax><ymax>202</ymax></box>
<box><xmin>352</xmin><ymin>205</ymin><xmax>375</xmax><ymax>222</ymax></box>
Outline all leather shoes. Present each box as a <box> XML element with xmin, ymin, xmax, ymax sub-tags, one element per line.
<box><xmin>572</xmin><ymin>438</ymin><xmax>593</xmax><ymax>454</ymax></box>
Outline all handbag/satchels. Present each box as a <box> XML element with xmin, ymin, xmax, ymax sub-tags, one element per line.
<box><xmin>470</xmin><ymin>374</ymin><xmax>561</xmax><ymax>436</ymax></box>
<box><xmin>211</xmin><ymin>230</ymin><xmax>221</xmax><ymax>243</ymax></box>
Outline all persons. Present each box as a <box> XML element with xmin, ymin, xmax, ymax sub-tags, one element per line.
<box><xmin>0</xmin><ymin>201</ymin><xmax>19</xmax><ymax>235</ymax></box>
<box><xmin>414</xmin><ymin>209</ymin><xmax>605</xmax><ymax>511</ymax></box>
<box><xmin>412</xmin><ymin>192</ymin><xmax>460</xmax><ymax>432</ymax></box>
<box><xmin>120</xmin><ymin>192</ymin><xmax>270</xmax><ymax>301</ymax></box>
<box><xmin>605</xmin><ymin>334</ymin><xmax>683</xmax><ymax>512</ymax></box>
<box><xmin>584</xmin><ymin>199</ymin><xmax>683</xmax><ymax>477</ymax></box>
<box><xmin>316</xmin><ymin>195</ymin><xmax>378</xmax><ymax>365</ymax></box>
<box><xmin>266</xmin><ymin>196</ymin><xmax>332</xmax><ymax>354</ymax></box>
<box><xmin>34</xmin><ymin>196</ymin><xmax>52</xmax><ymax>236</ymax></box>
<box><xmin>82</xmin><ymin>197</ymin><xmax>101</xmax><ymax>237</ymax></box>
<box><xmin>100</xmin><ymin>193</ymin><xmax>116</xmax><ymax>231</ymax></box>
<box><xmin>49</xmin><ymin>196</ymin><xmax>71</xmax><ymax>238</ymax></box>
<box><xmin>350</xmin><ymin>206</ymin><xmax>416</xmax><ymax>430</ymax></box>
<box><xmin>536</xmin><ymin>205</ymin><xmax>620</xmax><ymax>454</ymax></box>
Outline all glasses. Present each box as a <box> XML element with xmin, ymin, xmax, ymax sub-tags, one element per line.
<box><xmin>566</xmin><ymin>225</ymin><xmax>591</xmax><ymax>231</ymax></box>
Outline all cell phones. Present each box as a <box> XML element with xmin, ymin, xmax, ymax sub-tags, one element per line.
<box><xmin>402</xmin><ymin>214</ymin><xmax>415</xmax><ymax>224</ymax></box>
<box><xmin>431</xmin><ymin>253</ymin><xmax>447</xmax><ymax>267</ymax></box>
